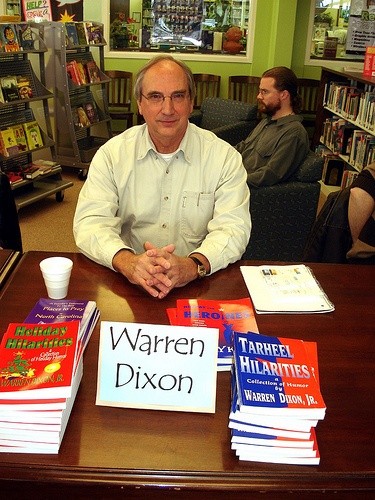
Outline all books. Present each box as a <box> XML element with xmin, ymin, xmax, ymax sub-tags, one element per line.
<box><xmin>0</xmin><ymin>299</ymin><xmax>100</xmax><ymax>454</ymax></box>
<box><xmin>0</xmin><ymin>121</ymin><xmax>43</xmax><ymax>157</ymax></box>
<box><xmin>7</xmin><ymin>159</ymin><xmax>61</xmax><ymax>187</ymax></box>
<box><xmin>227</xmin><ymin>330</ymin><xmax>326</xmax><ymax>466</ymax></box>
<box><xmin>167</xmin><ymin>297</ymin><xmax>260</xmax><ymax>372</ymax></box>
<box><xmin>65</xmin><ymin>22</ymin><xmax>103</xmax><ymax>46</ymax></box>
<box><xmin>0</xmin><ymin>24</ymin><xmax>35</xmax><ymax>53</ymax></box>
<box><xmin>66</xmin><ymin>59</ymin><xmax>100</xmax><ymax>86</ymax></box>
<box><xmin>319</xmin><ymin>81</ymin><xmax>375</xmax><ymax>190</ymax></box>
<box><xmin>240</xmin><ymin>265</ymin><xmax>336</xmax><ymax>314</ymax></box>
<box><xmin>0</xmin><ymin>75</ymin><xmax>34</xmax><ymax>103</ymax></box>
<box><xmin>71</xmin><ymin>102</ymin><xmax>98</xmax><ymax>131</ymax></box>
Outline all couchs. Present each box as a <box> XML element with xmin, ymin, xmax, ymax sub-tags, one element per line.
<box><xmin>190</xmin><ymin>96</ymin><xmax>259</xmax><ymax>144</ymax></box>
<box><xmin>239</xmin><ymin>149</ymin><xmax>325</xmax><ymax>260</ymax></box>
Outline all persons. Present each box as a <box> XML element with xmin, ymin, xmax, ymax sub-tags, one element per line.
<box><xmin>191</xmin><ymin>15</ymin><xmax>202</xmax><ymax>30</ymax></box>
<box><xmin>73</xmin><ymin>53</ymin><xmax>251</xmax><ymax>299</ymax></box>
<box><xmin>347</xmin><ymin>162</ymin><xmax>375</xmax><ymax>265</ymax></box>
<box><xmin>158</xmin><ymin>13</ymin><xmax>174</xmax><ymax>33</ymax></box>
<box><xmin>118</xmin><ymin>10</ymin><xmax>127</xmax><ymax>23</ymax></box>
<box><xmin>235</xmin><ymin>67</ymin><xmax>309</xmax><ymax>190</ymax></box>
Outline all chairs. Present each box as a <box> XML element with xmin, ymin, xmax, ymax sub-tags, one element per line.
<box><xmin>191</xmin><ymin>73</ymin><xmax>320</xmax><ymax>142</ymax></box>
<box><xmin>105</xmin><ymin>70</ymin><xmax>134</xmax><ymax>132</ymax></box>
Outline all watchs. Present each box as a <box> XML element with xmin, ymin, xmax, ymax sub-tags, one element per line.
<box><xmin>190</xmin><ymin>256</ymin><xmax>206</xmax><ymax>277</ymax></box>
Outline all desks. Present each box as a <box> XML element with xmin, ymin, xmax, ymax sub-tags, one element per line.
<box><xmin>0</xmin><ymin>250</ymin><xmax>375</xmax><ymax>500</ymax></box>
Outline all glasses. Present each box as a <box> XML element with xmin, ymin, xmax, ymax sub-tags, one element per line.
<box><xmin>140</xmin><ymin>92</ymin><xmax>190</xmax><ymax>104</ymax></box>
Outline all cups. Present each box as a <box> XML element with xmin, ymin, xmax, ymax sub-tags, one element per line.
<box><xmin>312</xmin><ymin>42</ymin><xmax>324</xmax><ymax>57</ymax></box>
<box><xmin>39</xmin><ymin>256</ymin><xmax>73</xmax><ymax>299</ymax></box>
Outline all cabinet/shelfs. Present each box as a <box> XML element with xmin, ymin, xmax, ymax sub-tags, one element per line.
<box><xmin>317</xmin><ymin>65</ymin><xmax>375</xmax><ymax>184</ymax></box>
